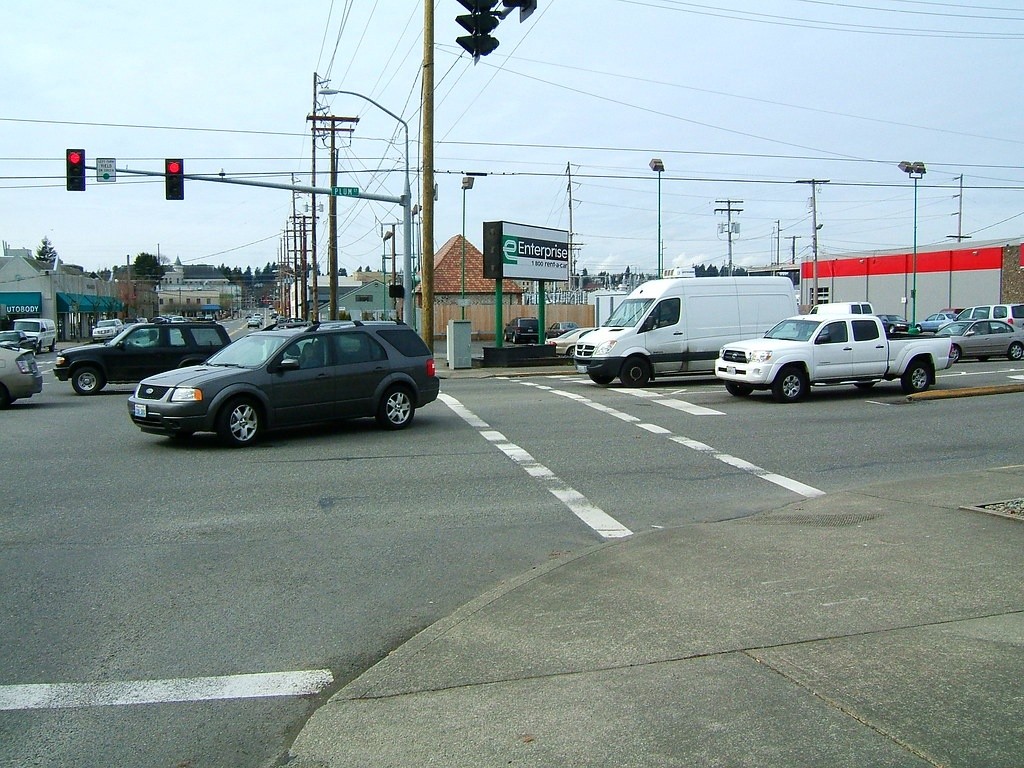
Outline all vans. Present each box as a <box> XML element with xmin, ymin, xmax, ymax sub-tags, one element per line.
<box><xmin>13</xmin><ymin>318</ymin><xmax>56</xmax><ymax>354</ymax></box>
<box><xmin>807</xmin><ymin>301</ymin><xmax>875</xmax><ymax>317</ymax></box>
<box><xmin>939</xmin><ymin>307</ymin><xmax>965</xmax><ymax>315</ymax></box>
<box><xmin>937</xmin><ymin>303</ymin><xmax>1024</xmax><ymax>330</ymax></box>
<box><xmin>572</xmin><ymin>275</ymin><xmax>800</xmax><ymax>388</ymax></box>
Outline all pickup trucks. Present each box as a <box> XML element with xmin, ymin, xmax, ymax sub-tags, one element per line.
<box><xmin>714</xmin><ymin>313</ymin><xmax>951</xmax><ymax>404</ymax></box>
<box><xmin>0</xmin><ymin>330</ymin><xmax>38</xmax><ymax>358</ymax></box>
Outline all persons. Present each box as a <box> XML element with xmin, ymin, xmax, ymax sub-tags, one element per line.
<box><xmin>129</xmin><ymin>330</ymin><xmax>158</xmax><ymax>347</ymax></box>
<box><xmin>653</xmin><ymin>303</ymin><xmax>674</xmax><ymax>327</ymax></box>
<box><xmin>298</xmin><ymin>342</ymin><xmax>322</xmax><ymax>368</ymax></box>
<box><xmin>823</xmin><ymin>324</ymin><xmax>843</xmax><ymax>341</ymax></box>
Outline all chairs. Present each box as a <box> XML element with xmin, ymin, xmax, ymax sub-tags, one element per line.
<box><xmin>286</xmin><ymin>344</ymin><xmax>300</xmax><ymax>360</ymax></box>
<box><xmin>311</xmin><ymin>341</ymin><xmax>324</xmax><ymax>365</ymax></box>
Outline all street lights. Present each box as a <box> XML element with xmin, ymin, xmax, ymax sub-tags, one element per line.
<box><xmin>411</xmin><ymin>203</ymin><xmax>423</xmax><ymax>332</ymax></box>
<box><xmin>272</xmin><ymin>270</ymin><xmax>298</xmax><ymax>322</ymax></box>
<box><xmin>647</xmin><ymin>158</ymin><xmax>666</xmax><ymax>324</ymax></box>
<box><xmin>382</xmin><ymin>230</ymin><xmax>392</xmax><ymax>321</ymax></box>
<box><xmin>317</xmin><ymin>89</ymin><xmax>412</xmax><ymax>328</ymax></box>
<box><xmin>897</xmin><ymin>160</ymin><xmax>927</xmax><ymax>335</ymax></box>
<box><xmin>461</xmin><ymin>176</ymin><xmax>475</xmax><ymax>320</ymax></box>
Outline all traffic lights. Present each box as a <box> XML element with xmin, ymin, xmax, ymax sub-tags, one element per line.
<box><xmin>165</xmin><ymin>158</ymin><xmax>184</xmax><ymax>200</ymax></box>
<box><xmin>66</xmin><ymin>148</ymin><xmax>86</xmax><ymax>191</ymax></box>
<box><xmin>455</xmin><ymin>0</ymin><xmax>500</xmax><ymax>61</ymax></box>
<box><xmin>501</xmin><ymin>0</ymin><xmax>537</xmax><ymax>23</ymax></box>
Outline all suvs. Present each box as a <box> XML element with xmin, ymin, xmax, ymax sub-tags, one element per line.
<box><xmin>92</xmin><ymin>319</ymin><xmax>124</xmax><ymax>343</ymax></box>
<box><xmin>504</xmin><ymin>317</ymin><xmax>538</xmax><ymax>344</ymax></box>
<box><xmin>53</xmin><ymin>317</ymin><xmax>232</xmax><ymax>396</ymax></box>
<box><xmin>127</xmin><ymin>320</ymin><xmax>440</xmax><ymax>448</ymax></box>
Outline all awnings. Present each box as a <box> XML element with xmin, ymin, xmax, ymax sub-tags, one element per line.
<box><xmin>101</xmin><ymin>296</ymin><xmax>120</xmax><ymax>312</ymax></box>
<box><xmin>113</xmin><ymin>296</ymin><xmax>124</xmax><ymax>311</ymax></box>
<box><xmin>84</xmin><ymin>295</ymin><xmax>109</xmax><ymax>312</ymax></box>
<box><xmin>57</xmin><ymin>292</ymin><xmax>78</xmax><ymax>313</ymax></box>
<box><xmin>67</xmin><ymin>294</ymin><xmax>94</xmax><ymax>313</ymax></box>
<box><xmin>0</xmin><ymin>292</ymin><xmax>42</xmax><ymax>314</ymax></box>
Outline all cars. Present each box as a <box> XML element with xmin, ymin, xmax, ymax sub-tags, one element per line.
<box><xmin>268</xmin><ymin>305</ymin><xmax>295</xmax><ymax>329</ymax></box>
<box><xmin>196</xmin><ymin>314</ymin><xmax>213</xmax><ymax>320</ymax></box>
<box><xmin>544</xmin><ymin>327</ymin><xmax>597</xmax><ymax>357</ymax></box>
<box><xmin>876</xmin><ymin>315</ymin><xmax>910</xmax><ymax>334</ymax></box>
<box><xmin>245</xmin><ymin>312</ymin><xmax>264</xmax><ymax>328</ymax></box>
<box><xmin>0</xmin><ymin>345</ymin><xmax>43</xmax><ymax>410</ymax></box>
<box><xmin>934</xmin><ymin>318</ymin><xmax>1024</xmax><ymax>363</ymax></box>
<box><xmin>545</xmin><ymin>322</ymin><xmax>579</xmax><ymax>340</ymax></box>
<box><xmin>124</xmin><ymin>313</ymin><xmax>186</xmax><ymax>330</ymax></box>
<box><xmin>915</xmin><ymin>312</ymin><xmax>958</xmax><ymax>333</ymax></box>
